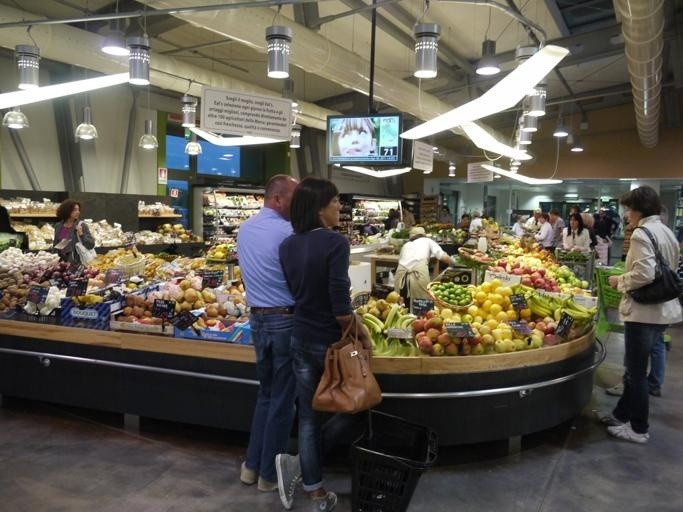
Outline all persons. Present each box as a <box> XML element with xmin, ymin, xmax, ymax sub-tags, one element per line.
<box><xmin>234</xmin><ymin>174</ymin><xmax>299</xmax><ymax>492</ymax></box>
<box><xmin>357</xmin><ymin>224</ymin><xmax>379</xmax><ymax>236</ymax></box>
<box><xmin>510</xmin><ymin>203</ymin><xmax>620</xmax><ymax>266</ymax></box>
<box><xmin>330</xmin><ymin>117</ymin><xmax>378</xmax><ymax>157</ymax></box>
<box><xmin>390</xmin><ymin>212</ymin><xmax>398</xmax><ymax>229</ymax></box>
<box><xmin>468</xmin><ymin>210</ymin><xmax>485</xmax><ymax>233</ymax></box>
<box><xmin>592</xmin><ymin>185</ymin><xmax>682</xmax><ymax>443</ymax></box>
<box><xmin>274</xmin><ymin>178</ymin><xmax>373</xmax><ymax>512</ymax></box>
<box><xmin>401</xmin><ymin>203</ymin><xmax>415</xmax><ymax>226</ymax></box>
<box><xmin>439</xmin><ymin>206</ymin><xmax>451</xmax><ymax>223</ymax></box>
<box><xmin>604</xmin><ymin>332</ymin><xmax>666</xmax><ymax>398</ymax></box>
<box><xmin>384</xmin><ymin>209</ymin><xmax>393</xmax><ymax>230</ymax></box>
<box><xmin>454</xmin><ymin>214</ymin><xmax>469</xmax><ymax>229</ymax></box>
<box><xmin>52</xmin><ymin>199</ymin><xmax>94</xmax><ymax>265</ymax></box>
<box><xmin>393</xmin><ymin>226</ymin><xmax>455</xmax><ymax>311</ymax></box>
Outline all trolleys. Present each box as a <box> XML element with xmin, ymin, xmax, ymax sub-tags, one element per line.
<box><xmin>555</xmin><ymin>248</ymin><xmax>596</xmax><ymax>281</ymax></box>
<box><xmin>592</xmin><ymin>259</ymin><xmax>671</xmax><ymax>355</ymax></box>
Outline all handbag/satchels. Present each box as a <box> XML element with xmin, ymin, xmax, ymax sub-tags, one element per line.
<box><xmin>624</xmin><ymin>225</ymin><xmax>683</xmax><ymax>306</ymax></box>
<box><xmin>74</xmin><ymin>220</ymin><xmax>98</xmax><ymax>266</ymax></box>
<box><xmin>309</xmin><ymin>311</ymin><xmax>383</xmax><ymax>418</ymax></box>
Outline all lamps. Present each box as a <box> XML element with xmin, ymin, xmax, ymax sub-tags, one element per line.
<box><xmin>71</xmin><ymin>23</ymin><xmax>100</xmax><ymax>143</ymax></box>
<box><xmin>97</xmin><ymin>19</ymin><xmax>130</xmax><ymax>60</ymax></box>
<box><xmin>1</xmin><ymin>106</ymin><xmax>30</xmax><ymax>131</ymax></box>
<box><xmin>508</xmin><ymin>40</ymin><xmax>583</xmax><ymax>177</ymax></box>
<box><xmin>176</xmin><ymin>76</ymin><xmax>308</xmax><ymax>159</ymax></box>
<box><xmin>407</xmin><ymin>3</ymin><xmax>444</xmax><ymax>82</ymax></box>
<box><xmin>136</xmin><ymin>88</ymin><xmax>163</xmax><ymax>152</ymax></box>
<box><xmin>261</xmin><ymin>2</ymin><xmax>297</xmax><ymax>83</ymax></box>
<box><xmin>125</xmin><ymin>16</ymin><xmax>155</xmax><ymax>88</ymax></box>
<box><xmin>326</xmin><ymin>143</ymin><xmax>461</xmax><ymax>178</ymax></box>
<box><xmin>474</xmin><ymin>1</ymin><xmax>505</xmax><ymax>78</ymax></box>
<box><xmin>12</xmin><ymin>26</ymin><xmax>43</xmax><ymax>91</ymax></box>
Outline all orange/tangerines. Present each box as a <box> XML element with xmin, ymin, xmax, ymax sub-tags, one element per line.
<box><xmin>431</xmin><ymin>281</ymin><xmax>472</xmax><ymax>307</ymax></box>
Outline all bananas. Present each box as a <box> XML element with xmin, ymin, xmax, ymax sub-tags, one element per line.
<box><xmin>524</xmin><ymin>287</ymin><xmax>599</xmax><ymax>326</ymax></box>
<box><xmin>361</xmin><ymin>303</ymin><xmax>421</xmax><ymax>357</ymax></box>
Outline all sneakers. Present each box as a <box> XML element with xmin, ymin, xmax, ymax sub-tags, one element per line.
<box><xmin>275</xmin><ymin>448</ymin><xmax>304</xmax><ymax>509</ymax></box>
<box><xmin>591</xmin><ymin>409</ymin><xmax>624</xmax><ymax>427</ymax></box>
<box><xmin>606</xmin><ymin>420</ymin><xmax>650</xmax><ymax>444</ymax></box>
<box><xmin>240</xmin><ymin>458</ymin><xmax>257</xmax><ymax>484</ymax></box>
<box><xmin>310</xmin><ymin>492</ymin><xmax>339</xmax><ymax>512</ymax></box>
<box><xmin>647</xmin><ymin>384</ymin><xmax>662</xmax><ymax>397</ymax></box>
<box><xmin>606</xmin><ymin>382</ymin><xmax>625</xmax><ymax>396</ymax></box>
<box><xmin>256</xmin><ymin>475</ymin><xmax>278</xmax><ymax>491</ymax></box>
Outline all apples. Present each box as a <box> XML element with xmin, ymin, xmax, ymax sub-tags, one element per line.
<box><xmin>411</xmin><ymin>305</ymin><xmax>531</xmax><ymax>358</ymax></box>
<box><xmin>487</xmin><ymin>259</ymin><xmax>561</xmax><ymax>293</ymax></box>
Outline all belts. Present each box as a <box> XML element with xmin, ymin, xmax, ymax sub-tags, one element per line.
<box><xmin>249</xmin><ymin>306</ymin><xmax>292</xmax><ymax>314</ymax></box>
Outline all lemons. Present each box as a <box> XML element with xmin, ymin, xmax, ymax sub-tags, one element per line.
<box><xmin>468</xmin><ymin>278</ymin><xmax>531</xmax><ymax>323</ymax></box>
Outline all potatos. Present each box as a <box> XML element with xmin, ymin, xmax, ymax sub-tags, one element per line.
<box><xmin>118</xmin><ymin>279</ymin><xmax>227</xmax><ymax>329</ymax></box>
<box><xmin>0</xmin><ymin>265</ymin><xmax>49</xmax><ymax>313</ymax></box>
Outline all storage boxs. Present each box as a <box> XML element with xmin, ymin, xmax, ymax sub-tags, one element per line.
<box><xmin>483</xmin><ymin>271</ymin><xmax>598</xmax><ymax>308</ymax></box>
<box><xmin>61</xmin><ymin>281</ymin><xmax>253</xmax><ymax>345</ymax></box>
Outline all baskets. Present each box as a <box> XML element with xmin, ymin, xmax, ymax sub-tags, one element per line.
<box><xmin>348</xmin><ymin>406</ymin><xmax>440</xmax><ymax>512</ymax></box>
<box><xmin>426</xmin><ymin>277</ymin><xmax>477</xmax><ymax>310</ymax></box>
<box><xmin>113</xmin><ymin>252</ymin><xmax>146</xmax><ymax>275</ymax></box>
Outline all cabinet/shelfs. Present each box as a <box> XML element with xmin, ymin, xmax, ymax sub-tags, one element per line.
<box><xmin>332</xmin><ymin>192</ymin><xmax>439</xmax><ymax>236</ymax></box>
<box><xmin>192</xmin><ymin>186</ymin><xmax>267</xmax><ymax>243</ymax></box>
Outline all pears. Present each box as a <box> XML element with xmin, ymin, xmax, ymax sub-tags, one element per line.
<box><xmin>356</xmin><ymin>291</ymin><xmax>401</xmax><ymax>319</ymax></box>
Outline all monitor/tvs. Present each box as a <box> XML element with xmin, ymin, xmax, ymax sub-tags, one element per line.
<box><xmin>326</xmin><ymin>113</ymin><xmax>403</xmax><ymax>164</ymax></box>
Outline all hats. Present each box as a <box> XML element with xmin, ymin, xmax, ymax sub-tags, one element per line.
<box><xmin>409</xmin><ymin>226</ymin><xmax>425</xmax><ymax>238</ymax></box>
